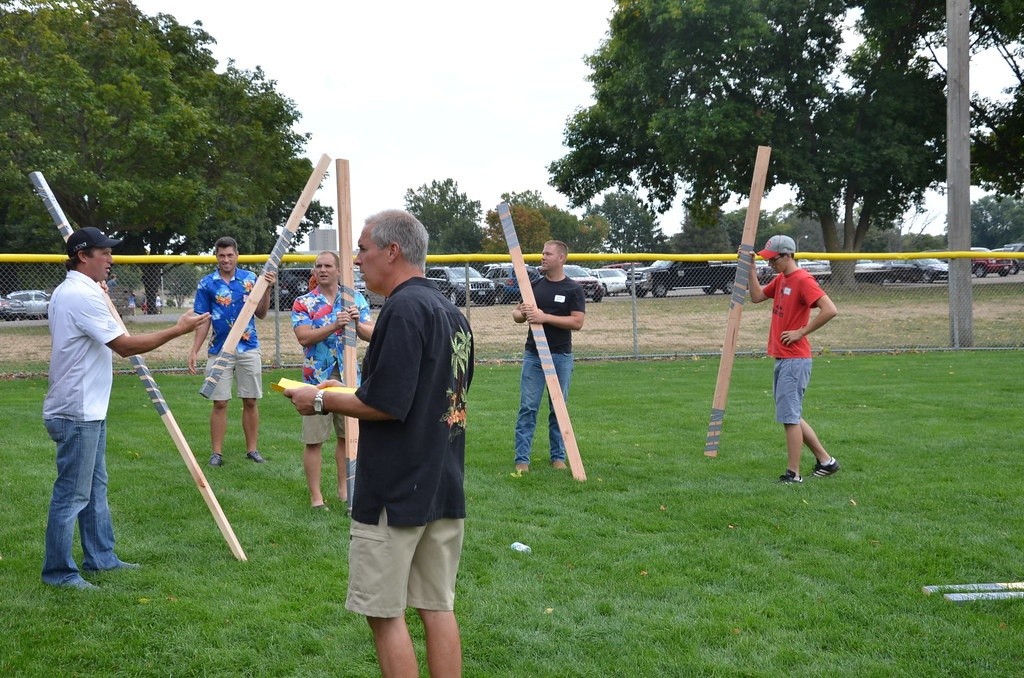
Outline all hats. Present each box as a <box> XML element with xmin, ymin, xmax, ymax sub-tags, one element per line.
<box><xmin>66</xmin><ymin>227</ymin><xmax>121</xmax><ymax>256</ymax></box>
<box><xmin>756</xmin><ymin>235</ymin><xmax>796</xmax><ymax>259</ymax></box>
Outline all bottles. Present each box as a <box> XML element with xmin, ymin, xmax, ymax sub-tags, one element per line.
<box><xmin>511</xmin><ymin>541</ymin><xmax>532</xmax><ymax>554</ymax></box>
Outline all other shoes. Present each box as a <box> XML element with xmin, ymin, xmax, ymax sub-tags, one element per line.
<box><xmin>553</xmin><ymin>459</ymin><xmax>567</xmax><ymax>470</ymax></box>
<box><xmin>516</xmin><ymin>462</ymin><xmax>528</xmax><ymax>471</ymax></box>
<box><xmin>64</xmin><ymin>579</ymin><xmax>99</xmax><ymax>592</ymax></box>
<box><xmin>313</xmin><ymin>504</ymin><xmax>329</xmax><ymax>511</ymax></box>
<box><xmin>115</xmin><ymin>559</ymin><xmax>140</xmax><ymax>569</ymax></box>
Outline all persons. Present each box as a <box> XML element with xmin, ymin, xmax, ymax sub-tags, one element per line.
<box><xmin>513</xmin><ymin>240</ymin><xmax>585</xmax><ymax>471</ymax></box>
<box><xmin>106</xmin><ymin>273</ymin><xmax>163</xmax><ymax>315</ymax></box>
<box><xmin>291</xmin><ymin>251</ymin><xmax>376</xmax><ymax>511</ymax></box>
<box><xmin>188</xmin><ymin>237</ymin><xmax>277</xmax><ymax>465</ymax></box>
<box><xmin>283</xmin><ymin>210</ymin><xmax>474</xmax><ymax>678</ymax></box>
<box><xmin>40</xmin><ymin>227</ymin><xmax>211</xmax><ymax>589</ymax></box>
<box><xmin>737</xmin><ymin>235</ymin><xmax>841</xmax><ymax>483</ymax></box>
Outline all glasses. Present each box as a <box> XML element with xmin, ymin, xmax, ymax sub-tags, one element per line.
<box><xmin>768</xmin><ymin>253</ymin><xmax>785</xmax><ymax>262</ymax></box>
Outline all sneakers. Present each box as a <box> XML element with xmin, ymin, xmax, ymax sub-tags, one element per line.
<box><xmin>773</xmin><ymin>469</ymin><xmax>803</xmax><ymax>485</ymax></box>
<box><xmin>247</xmin><ymin>450</ymin><xmax>263</xmax><ymax>463</ymax></box>
<box><xmin>810</xmin><ymin>456</ymin><xmax>841</xmax><ymax>477</ymax></box>
<box><xmin>210</xmin><ymin>453</ymin><xmax>222</xmax><ymax>466</ymax></box>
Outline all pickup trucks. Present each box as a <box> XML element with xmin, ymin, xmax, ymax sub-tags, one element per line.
<box><xmin>624</xmin><ymin>260</ymin><xmax>740</xmax><ymax>298</ymax></box>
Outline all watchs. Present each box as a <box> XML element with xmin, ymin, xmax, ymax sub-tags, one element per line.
<box><xmin>313</xmin><ymin>389</ymin><xmax>327</xmax><ymax>415</ymax></box>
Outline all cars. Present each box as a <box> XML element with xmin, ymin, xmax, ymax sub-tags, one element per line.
<box><xmin>753</xmin><ymin>258</ymin><xmax>949</xmax><ymax>285</ymax></box>
<box><xmin>969</xmin><ymin>243</ymin><xmax>1024</xmax><ymax>277</ymax></box>
<box><xmin>481</xmin><ymin>263</ymin><xmax>627</xmax><ymax>303</ymax></box>
<box><xmin>352</xmin><ymin>269</ymin><xmax>367</xmax><ymax>295</ymax></box>
<box><xmin>145</xmin><ymin>295</ymin><xmax>161</xmax><ymax>313</ymax></box>
<box><xmin>0</xmin><ymin>290</ymin><xmax>52</xmax><ymax>322</ymax></box>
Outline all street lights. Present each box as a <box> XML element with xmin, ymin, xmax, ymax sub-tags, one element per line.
<box><xmin>797</xmin><ymin>235</ymin><xmax>810</xmax><ymax>252</ymax></box>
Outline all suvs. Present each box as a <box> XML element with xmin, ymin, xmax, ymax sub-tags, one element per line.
<box><xmin>425</xmin><ymin>266</ymin><xmax>496</xmax><ymax>306</ymax></box>
<box><xmin>269</xmin><ymin>267</ymin><xmax>313</xmax><ymax>310</ymax></box>
